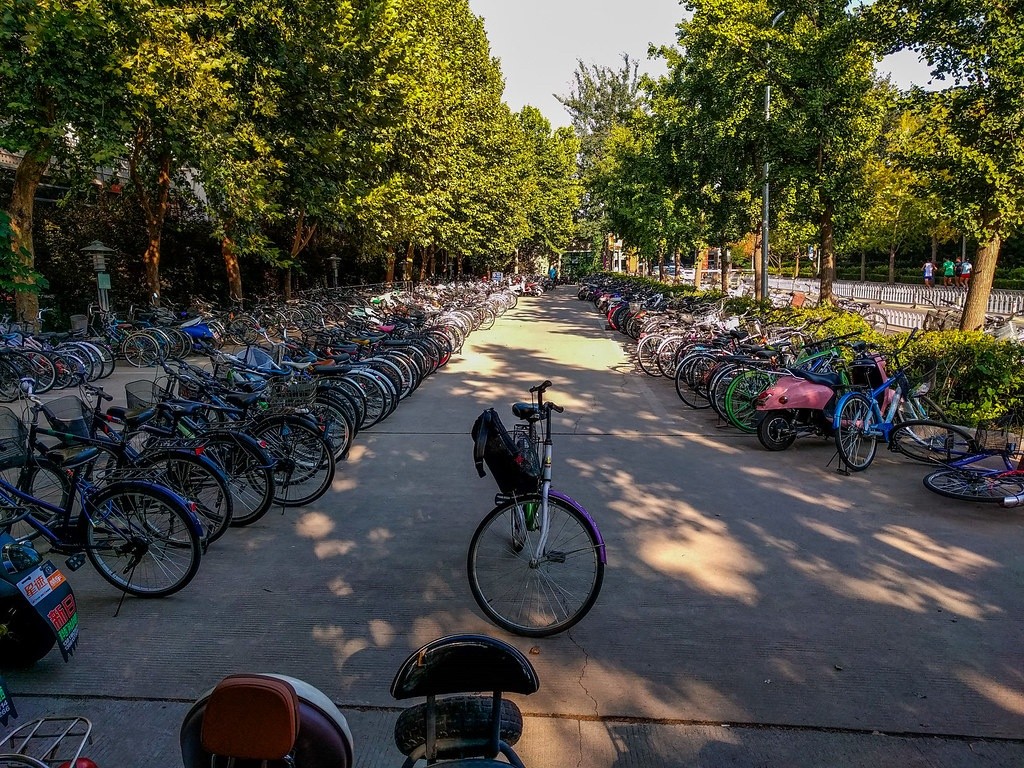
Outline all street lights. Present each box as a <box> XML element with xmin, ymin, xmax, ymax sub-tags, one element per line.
<box><xmin>399</xmin><ymin>259</ymin><xmax>410</xmax><ymax>289</ymax></box>
<box><xmin>81</xmin><ymin>239</ymin><xmax>117</xmax><ymax>326</ymax></box>
<box><xmin>328</xmin><ymin>253</ymin><xmax>341</xmax><ymax>289</ymax></box>
<box><xmin>447</xmin><ymin>262</ymin><xmax>454</xmax><ymax>280</ymax></box>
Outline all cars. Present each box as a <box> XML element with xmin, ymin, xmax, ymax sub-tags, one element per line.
<box><xmin>638</xmin><ymin>258</ymin><xmax>750</xmax><ymax>297</ymax></box>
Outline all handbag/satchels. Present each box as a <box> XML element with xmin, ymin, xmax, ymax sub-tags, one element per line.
<box><xmin>472</xmin><ymin>407</ymin><xmax>539</xmax><ymax>494</ymax></box>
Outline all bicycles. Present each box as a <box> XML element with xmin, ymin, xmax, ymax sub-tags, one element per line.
<box><xmin>887</xmin><ymin>411</ymin><xmax>1024</xmax><ymax>510</ymax></box>
<box><xmin>560</xmin><ymin>273</ymin><xmax>578</xmax><ymax>287</ymax></box>
<box><xmin>578</xmin><ymin>272</ymin><xmax>954</xmax><ymax>477</ymax></box>
<box><xmin>1</xmin><ymin>390</ymin><xmax>538</xmax><ymax>768</ymax></box>
<box><xmin>465</xmin><ymin>381</ymin><xmax>610</xmax><ymax>640</ymax></box>
<box><xmin>955</xmin><ymin>302</ymin><xmax>1024</xmax><ymax>345</ymax></box>
<box><xmin>1</xmin><ymin>269</ymin><xmax>560</xmax><ymax>391</ymax></box>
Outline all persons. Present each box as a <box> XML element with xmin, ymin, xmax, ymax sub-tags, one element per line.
<box><xmin>942</xmin><ymin>256</ymin><xmax>956</xmax><ymax>289</ymax></box>
<box><xmin>920</xmin><ymin>256</ymin><xmax>937</xmax><ymax>288</ymax></box>
<box><xmin>550</xmin><ymin>266</ymin><xmax>556</xmax><ymax>281</ymax></box>
<box><xmin>954</xmin><ymin>257</ymin><xmax>962</xmax><ymax>288</ymax></box>
<box><xmin>959</xmin><ymin>257</ymin><xmax>972</xmax><ymax>291</ymax></box>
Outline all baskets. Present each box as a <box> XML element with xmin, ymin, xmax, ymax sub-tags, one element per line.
<box><xmin>0</xmin><ymin>405</ymin><xmax>29</xmax><ymax>471</ymax></box>
<box><xmin>236</xmin><ymin>347</ymin><xmax>273</xmax><ymax>369</ymax></box>
<box><xmin>70</xmin><ymin>314</ymin><xmax>88</xmax><ymax>334</ymax></box>
<box><xmin>125</xmin><ymin>379</ymin><xmax>171</xmax><ymax>409</ymax></box>
<box><xmin>265</xmin><ymin>375</ymin><xmax>318</xmax><ymax>414</ymax></box>
<box><xmin>900</xmin><ymin>357</ymin><xmax>938</xmax><ymax>399</ymax></box>
<box><xmin>922</xmin><ymin>310</ymin><xmax>1017</xmax><ymax>343</ymax></box>
<box><xmin>178</xmin><ymin>365</ymin><xmax>212</xmax><ymax>400</ymax></box>
<box><xmin>43</xmin><ymin>395</ymin><xmax>93</xmax><ymax>443</ymax></box>
<box><xmin>676</xmin><ymin>300</ymin><xmax>790</xmax><ymax>345</ymax></box>
